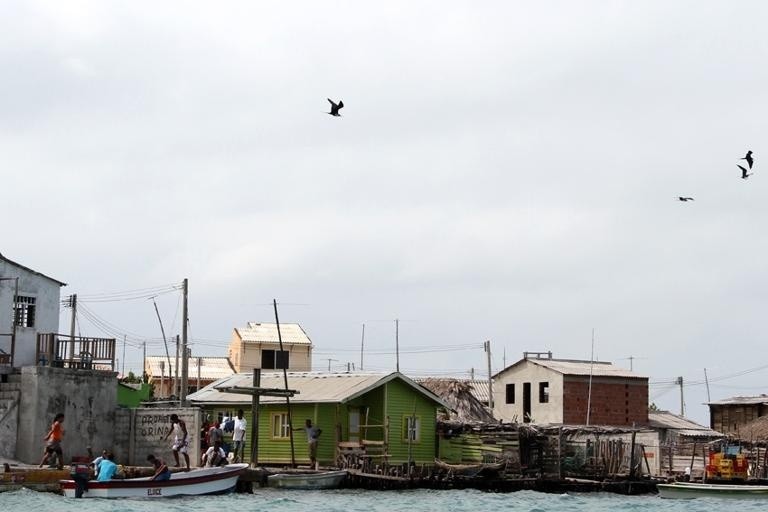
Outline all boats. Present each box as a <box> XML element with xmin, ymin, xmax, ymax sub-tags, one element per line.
<box><xmin>432</xmin><ymin>458</ymin><xmax>506</xmax><ymax>475</ymax></box>
<box><xmin>656</xmin><ymin>483</ymin><xmax>768</xmax><ymax>500</ymax></box>
<box><xmin>59</xmin><ymin>463</ymin><xmax>248</xmax><ymax>500</ymax></box>
<box><xmin>0</xmin><ymin>463</ymin><xmax>71</xmax><ymax>494</ymax></box>
<box><xmin>267</xmin><ymin>470</ymin><xmax>348</xmax><ymax>489</ymax></box>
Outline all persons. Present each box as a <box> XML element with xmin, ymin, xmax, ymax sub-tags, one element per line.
<box><xmin>147</xmin><ymin>455</ymin><xmax>170</xmax><ymax>480</ymax></box>
<box><xmin>39</xmin><ymin>414</ymin><xmax>65</xmax><ymax>469</ymax></box>
<box><xmin>96</xmin><ymin>453</ymin><xmax>117</xmax><ymax>481</ymax></box>
<box><xmin>89</xmin><ymin>450</ymin><xmax>107</xmax><ymax>479</ymax></box>
<box><xmin>201</xmin><ymin>409</ymin><xmax>248</xmax><ymax>467</ymax></box>
<box><xmin>292</xmin><ymin>419</ymin><xmax>322</xmax><ymax>469</ymax></box>
<box><xmin>164</xmin><ymin>414</ymin><xmax>189</xmax><ymax>471</ymax></box>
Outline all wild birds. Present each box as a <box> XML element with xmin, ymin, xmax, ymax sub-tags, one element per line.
<box><xmin>736</xmin><ymin>164</ymin><xmax>754</xmax><ymax>179</ymax></box>
<box><xmin>741</xmin><ymin>150</ymin><xmax>754</xmax><ymax>169</ymax></box>
<box><xmin>327</xmin><ymin>98</ymin><xmax>344</xmax><ymax>117</ymax></box>
<box><xmin>672</xmin><ymin>197</ymin><xmax>694</xmax><ymax>202</ymax></box>
